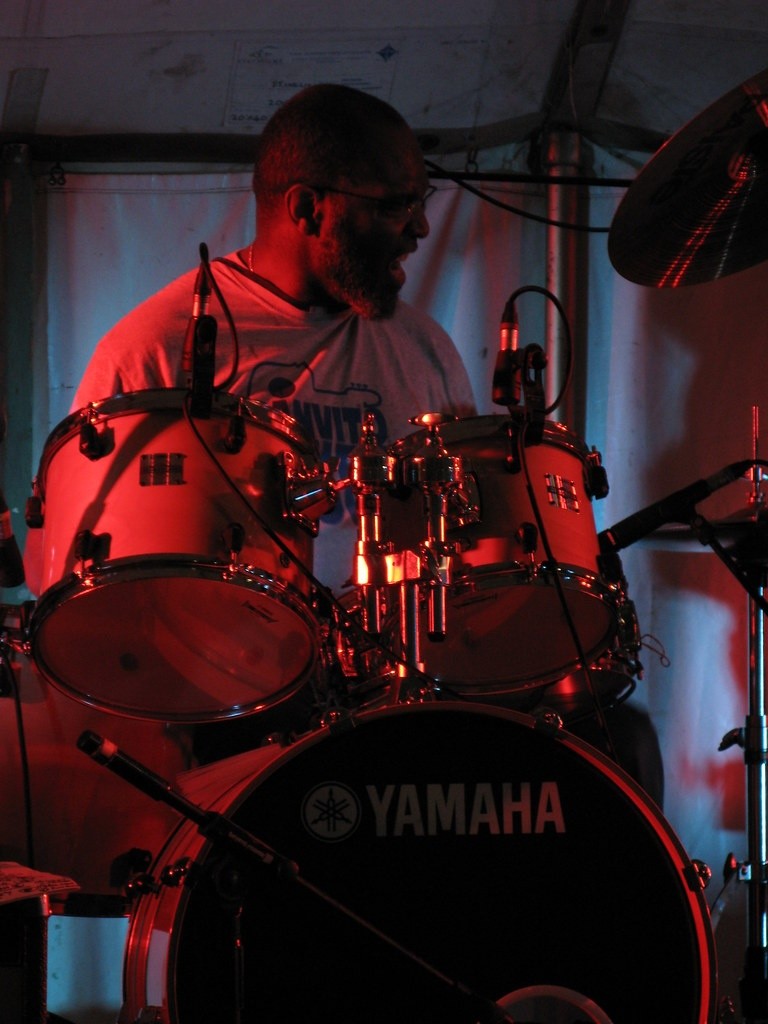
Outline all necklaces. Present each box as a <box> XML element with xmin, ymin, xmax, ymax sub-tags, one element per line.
<box><xmin>249</xmin><ymin>243</ymin><xmax>253</xmax><ymax>272</ymax></box>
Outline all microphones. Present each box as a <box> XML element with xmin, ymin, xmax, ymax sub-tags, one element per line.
<box><xmin>597</xmin><ymin>460</ymin><xmax>755</xmax><ymax>554</ymax></box>
<box><xmin>180</xmin><ymin>269</ymin><xmax>212</xmax><ymax>374</ymax></box>
<box><xmin>491</xmin><ymin>301</ymin><xmax>520</xmax><ymax>406</ymax></box>
<box><xmin>0</xmin><ymin>493</ymin><xmax>25</xmax><ymax>588</ymax></box>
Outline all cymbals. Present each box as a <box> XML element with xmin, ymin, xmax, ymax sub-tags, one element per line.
<box><xmin>641</xmin><ymin>499</ymin><xmax>768</xmax><ymax>543</ymax></box>
<box><xmin>607</xmin><ymin>67</ymin><xmax>768</xmax><ymax>290</ymax></box>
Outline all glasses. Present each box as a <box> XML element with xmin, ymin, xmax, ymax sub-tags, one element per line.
<box><xmin>306</xmin><ymin>184</ymin><xmax>438</xmax><ymax>223</ymax></box>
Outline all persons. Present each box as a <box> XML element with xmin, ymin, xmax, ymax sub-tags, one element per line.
<box><xmin>69</xmin><ymin>80</ymin><xmax>664</xmax><ymax>810</ymax></box>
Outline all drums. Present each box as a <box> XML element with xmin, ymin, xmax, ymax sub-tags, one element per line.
<box><xmin>353</xmin><ymin>413</ymin><xmax>624</xmax><ymax>699</ymax></box>
<box><xmin>18</xmin><ymin>383</ymin><xmax>344</xmax><ymax>726</ymax></box>
<box><xmin>117</xmin><ymin>697</ymin><xmax>720</xmax><ymax>1024</ymax></box>
<box><xmin>0</xmin><ymin>597</ymin><xmax>225</xmax><ymax>920</ymax></box>
